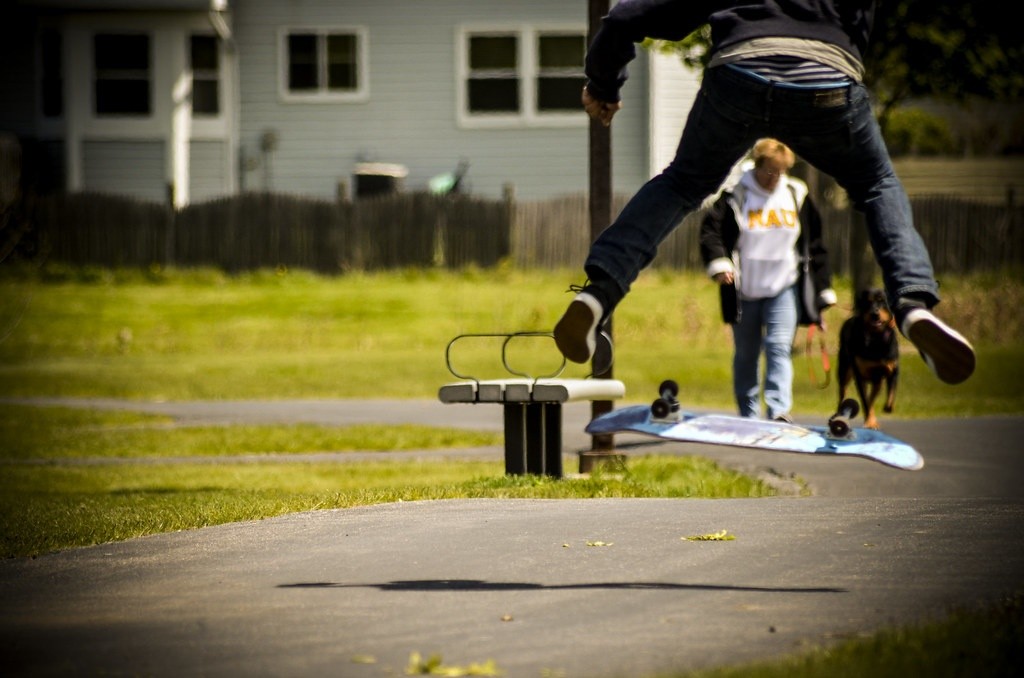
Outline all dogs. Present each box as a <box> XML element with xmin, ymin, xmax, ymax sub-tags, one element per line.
<box><xmin>837</xmin><ymin>289</ymin><xmax>899</xmax><ymax>429</ymax></box>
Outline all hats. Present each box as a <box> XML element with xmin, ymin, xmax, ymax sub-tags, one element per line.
<box><xmin>752</xmin><ymin>138</ymin><xmax>795</xmax><ymax>169</ymax></box>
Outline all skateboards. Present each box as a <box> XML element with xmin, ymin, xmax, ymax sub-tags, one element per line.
<box><xmin>585</xmin><ymin>377</ymin><xmax>924</xmax><ymax>473</ymax></box>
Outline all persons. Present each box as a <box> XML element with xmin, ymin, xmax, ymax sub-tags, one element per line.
<box><xmin>696</xmin><ymin>137</ymin><xmax>837</xmax><ymax>422</ymax></box>
<box><xmin>546</xmin><ymin>0</ymin><xmax>976</xmax><ymax>386</ymax></box>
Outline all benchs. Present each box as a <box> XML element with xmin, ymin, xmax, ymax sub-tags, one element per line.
<box><xmin>439</xmin><ymin>328</ymin><xmax>626</xmax><ymax>479</ymax></box>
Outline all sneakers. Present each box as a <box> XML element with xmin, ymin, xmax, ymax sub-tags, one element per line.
<box><xmin>553</xmin><ymin>278</ymin><xmax>615</xmax><ymax>364</ymax></box>
<box><xmin>893</xmin><ymin>299</ymin><xmax>978</xmax><ymax>387</ymax></box>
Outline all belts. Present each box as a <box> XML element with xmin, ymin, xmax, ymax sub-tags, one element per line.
<box><xmin>718</xmin><ymin>66</ymin><xmax>849</xmax><ymax>109</ymax></box>
